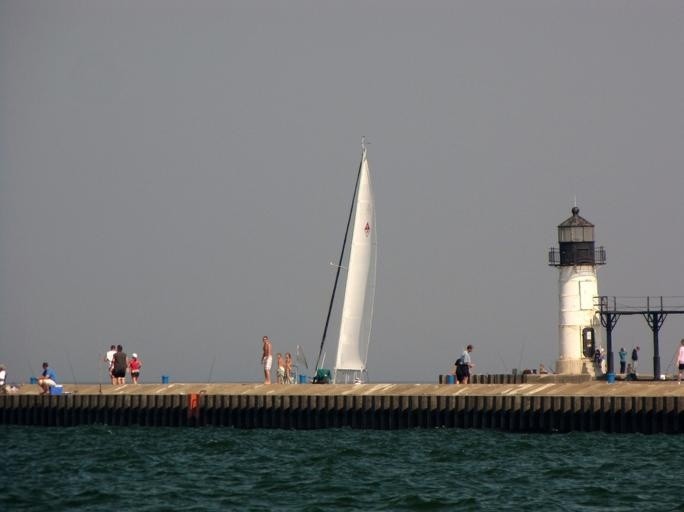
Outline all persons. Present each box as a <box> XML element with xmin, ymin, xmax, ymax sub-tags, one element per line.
<box><xmin>594</xmin><ymin>349</ymin><xmax>602</xmax><ymax>368</ymax></box>
<box><xmin>284</xmin><ymin>351</ymin><xmax>295</xmax><ymax>384</ymax></box>
<box><xmin>674</xmin><ymin>339</ymin><xmax>684</xmax><ymax>384</ymax></box>
<box><xmin>455</xmin><ymin>344</ymin><xmax>475</xmax><ymax>384</ymax></box>
<box><xmin>260</xmin><ymin>335</ymin><xmax>273</xmax><ymax>384</ymax></box>
<box><xmin>108</xmin><ymin>344</ymin><xmax>129</xmax><ymax>384</ymax></box>
<box><xmin>627</xmin><ymin>362</ymin><xmax>640</xmax><ymax>379</ymax></box>
<box><xmin>103</xmin><ymin>345</ymin><xmax>119</xmax><ymax>386</ymax></box>
<box><xmin>275</xmin><ymin>353</ymin><xmax>287</xmax><ymax>384</ymax></box>
<box><xmin>0</xmin><ymin>364</ymin><xmax>8</xmax><ymax>386</ymax></box>
<box><xmin>618</xmin><ymin>347</ymin><xmax>629</xmax><ymax>374</ymax></box>
<box><xmin>631</xmin><ymin>345</ymin><xmax>641</xmax><ymax>370</ymax></box>
<box><xmin>35</xmin><ymin>360</ymin><xmax>60</xmax><ymax>395</ymax></box>
<box><xmin>127</xmin><ymin>352</ymin><xmax>142</xmax><ymax>384</ymax></box>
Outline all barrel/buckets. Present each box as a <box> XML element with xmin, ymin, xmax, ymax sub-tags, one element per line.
<box><xmin>31</xmin><ymin>377</ymin><xmax>37</xmax><ymax>384</ymax></box>
<box><xmin>162</xmin><ymin>376</ymin><xmax>168</xmax><ymax>384</ymax></box>
<box><xmin>607</xmin><ymin>372</ymin><xmax>615</xmax><ymax>383</ymax></box>
<box><xmin>447</xmin><ymin>375</ymin><xmax>455</xmax><ymax>384</ymax></box>
<box><xmin>299</xmin><ymin>374</ymin><xmax>305</xmax><ymax>383</ymax></box>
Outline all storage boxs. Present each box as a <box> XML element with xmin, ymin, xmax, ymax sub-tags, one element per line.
<box><xmin>49</xmin><ymin>384</ymin><xmax>63</xmax><ymax>395</ymax></box>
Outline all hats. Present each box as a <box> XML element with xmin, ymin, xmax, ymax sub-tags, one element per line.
<box><xmin>132</xmin><ymin>353</ymin><xmax>138</xmax><ymax>358</ymax></box>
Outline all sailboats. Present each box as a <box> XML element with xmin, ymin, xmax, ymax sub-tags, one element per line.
<box><xmin>306</xmin><ymin>134</ymin><xmax>377</xmax><ymax>386</ymax></box>
